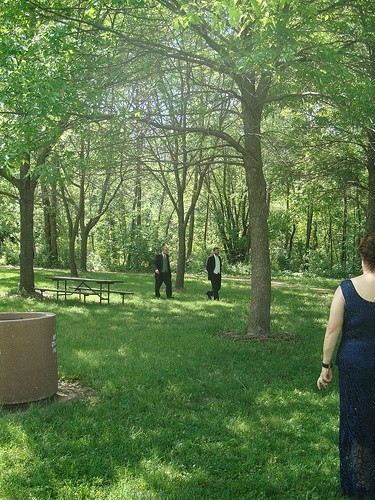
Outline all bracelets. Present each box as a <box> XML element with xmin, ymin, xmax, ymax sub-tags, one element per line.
<box><xmin>321</xmin><ymin>361</ymin><xmax>333</xmax><ymax>368</ymax></box>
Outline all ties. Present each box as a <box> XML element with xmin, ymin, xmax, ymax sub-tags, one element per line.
<box><xmin>164</xmin><ymin>254</ymin><xmax>166</xmax><ymax>259</ymax></box>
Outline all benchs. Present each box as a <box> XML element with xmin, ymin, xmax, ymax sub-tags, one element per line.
<box><xmin>33</xmin><ymin>287</ymin><xmax>99</xmax><ymax>304</ymax></box>
<box><xmin>69</xmin><ymin>286</ymin><xmax>134</xmax><ymax>304</ymax></box>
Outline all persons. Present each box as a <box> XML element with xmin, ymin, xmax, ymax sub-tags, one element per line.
<box><xmin>205</xmin><ymin>247</ymin><xmax>222</xmax><ymax>302</ymax></box>
<box><xmin>154</xmin><ymin>246</ymin><xmax>175</xmax><ymax>299</ymax></box>
<box><xmin>317</xmin><ymin>233</ymin><xmax>375</xmax><ymax>500</ymax></box>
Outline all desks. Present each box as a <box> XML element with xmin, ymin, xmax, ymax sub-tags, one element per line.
<box><xmin>43</xmin><ymin>275</ymin><xmax>125</xmax><ymax>304</ymax></box>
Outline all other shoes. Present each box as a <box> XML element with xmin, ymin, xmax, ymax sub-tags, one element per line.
<box><xmin>154</xmin><ymin>294</ymin><xmax>161</xmax><ymax>298</ymax></box>
<box><xmin>206</xmin><ymin>291</ymin><xmax>212</xmax><ymax>299</ymax></box>
<box><xmin>166</xmin><ymin>295</ymin><xmax>174</xmax><ymax>299</ymax></box>
<box><xmin>214</xmin><ymin>297</ymin><xmax>219</xmax><ymax>301</ymax></box>
